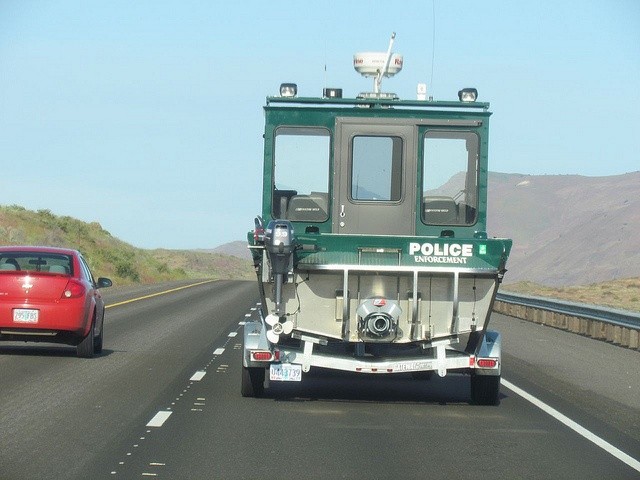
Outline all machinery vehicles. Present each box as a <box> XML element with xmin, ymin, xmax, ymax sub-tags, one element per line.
<box><xmin>242</xmin><ymin>33</ymin><xmax>512</xmax><ymax>403</ymax></box>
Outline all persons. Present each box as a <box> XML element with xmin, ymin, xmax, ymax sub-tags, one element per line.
<box><xmin>6</xmin><ymin>258</ymin><xmax>21</xmax><ymax>271</ymax></box>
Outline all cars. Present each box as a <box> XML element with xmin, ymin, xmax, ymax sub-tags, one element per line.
<box><xmin>0</xmin><ymin>246</ymin><xmax>112</xmax><ymax>357</ymax></box>
